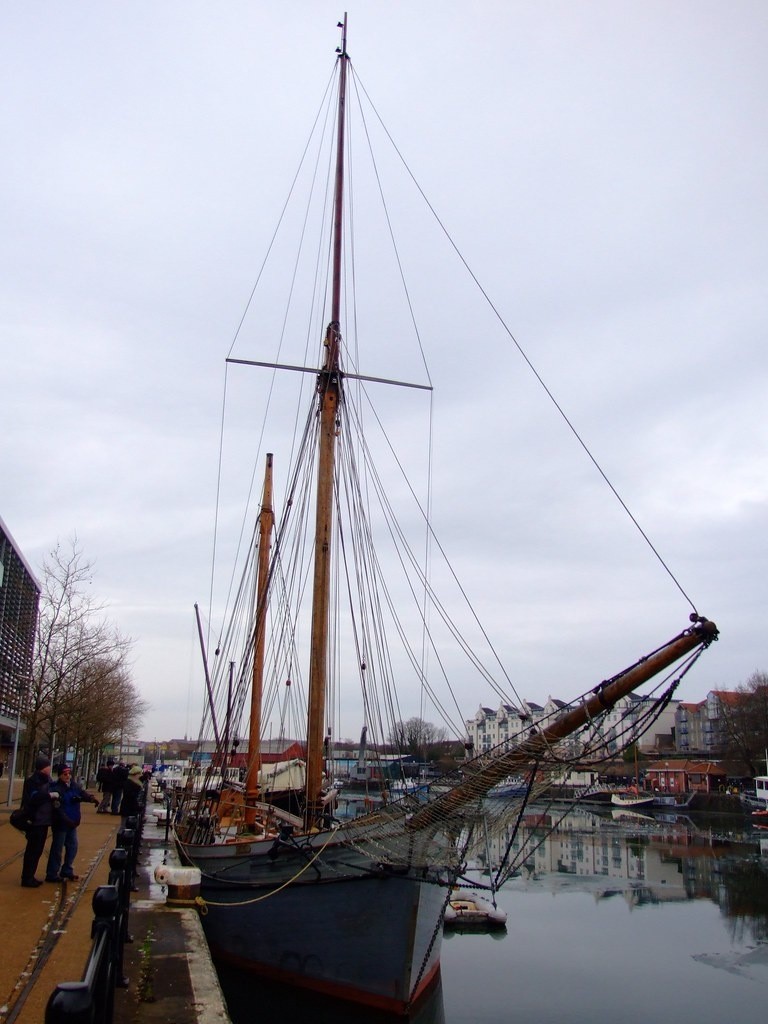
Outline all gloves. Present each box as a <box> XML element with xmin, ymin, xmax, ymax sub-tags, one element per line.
<box><xmin>90</xmin><ymin>797</ymin><xmax>99</xmax><ymax>808</ymax></box>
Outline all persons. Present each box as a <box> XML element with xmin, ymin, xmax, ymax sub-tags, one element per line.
<box><xmin>20</xmin><ymin>757</ymin><xmax>61</xmax><ymax>888</ymax></box>
<box><xmin>96</xmin><ymin>757</ymin><xmax>150</xmax><ymax>815</ymax></box>
<box><xmin>44</xmin><ymin>762</ymin><xmax>98</xmax><ymax>883</ymax></box>
<box><xmin>115</xmin><ymin>764</ymin><xmax>145</xmax><ymax>848</ymax></box>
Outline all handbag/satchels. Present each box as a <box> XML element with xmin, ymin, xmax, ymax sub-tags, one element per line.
<box><xmin>10</xmin><ymin>809</ymin><xmax>26</xmax><ymax>832</ymax></box>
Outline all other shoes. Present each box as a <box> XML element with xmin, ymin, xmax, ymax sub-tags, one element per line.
<box><xmin>97</xmin><ymin>810</ymin><xmax>111</xmax><ymax>815</ymax></box>
<box><xmin>21</xmin><ymin>877</ymin><xmax>43</xmax><ymax>888</ymax></box>
<box><xmin>44</xmin><ymin>874</ymin><xmax>64</xmax><ymax>883</ymax></box>
<box><xmin>110</xmin><ymin>811</ymin><xmax>121</xmax><ymax>816</ymax></box>
<box><xmin>59</xmin><ymin>870</ymin><xmax>79</xmax><ymax>882</ymax></box>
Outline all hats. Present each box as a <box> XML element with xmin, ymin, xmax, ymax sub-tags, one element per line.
<box><xmin>128</xmin><ymin>766</ymin><xmax>143</xmax><ymax>775</ymax></box>
<box><xmin>57</xmin><ymin>763</ymin><xmax>72</xmax><ymax>777</ymax></box>
<box><xmin>35</xmin><ymin>755</ymin><xmax>51</xmax><ymax>771</ymax></box>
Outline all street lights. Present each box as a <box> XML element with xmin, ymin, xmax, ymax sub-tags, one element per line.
<box><xmin>7</xmin><ymin>673</ymin><xmax>28</xmax><ymax>807</ymax></box>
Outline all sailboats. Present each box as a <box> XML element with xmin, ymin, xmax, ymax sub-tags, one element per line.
<box><xmin>610</xmin><ymin>731</ymin><xmax>654</xmax><ymax>807</ymax></box>
<box><xmin>163</xmin><ymin>5</ymin><xmax>720</xmax><ymax>1023</ymax></box>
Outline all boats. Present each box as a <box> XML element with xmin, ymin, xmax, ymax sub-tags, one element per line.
<box><xmin>388</xmin><ymin>777</ymin><xmax>431</xmax><ymax>793</ymax></box>
<box><xmin>487</xmin><ymin>775</ymin><xmax>527</xmax><ymax>799</ymax></box>
<box><xmin>751</xmin><ymin>810</ymin><xmax>768</xmax><ymax>818</ymax></box>
<box><xmin>444</xmin><ymin>889</ymin><xmax>509</xmax><ymax>928</ymax></box>
<box><xmin>156</xmin><ymin>766</ymin><xmax>240</xmax><ymax>794</ymax></box>
<box><xmin>327</xmin><ymin>777</ymin><xmax>344</xmax><ymax>792</ymax></box>
